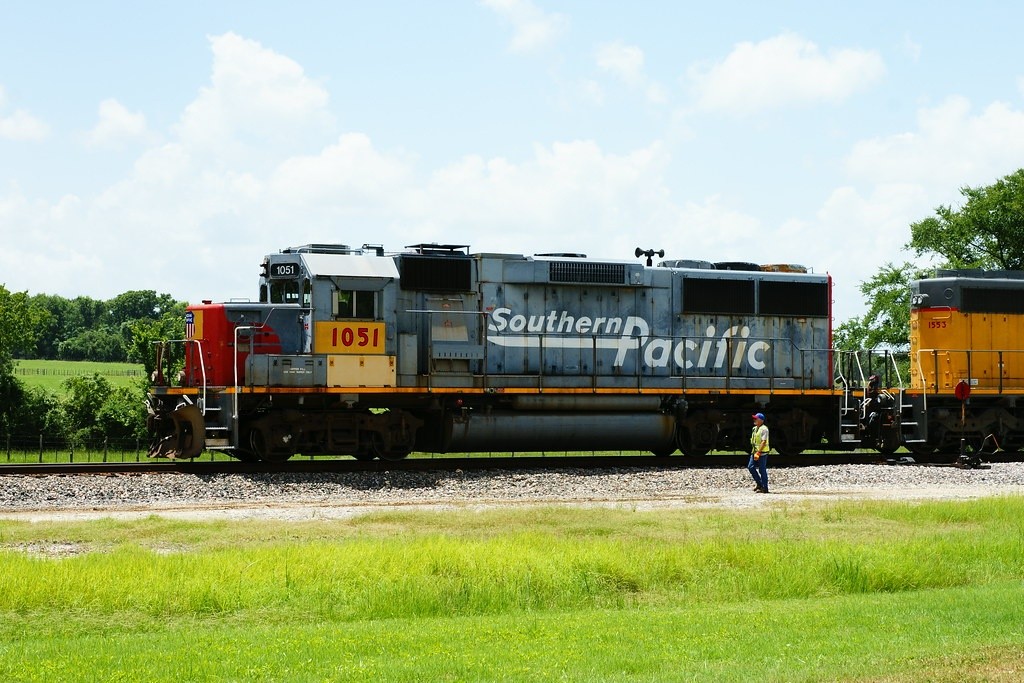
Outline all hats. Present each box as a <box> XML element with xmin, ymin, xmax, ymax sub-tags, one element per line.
<box><xmin>752</xmin><ymin>413</ymin><xmax>765</xmax><ymax>420</ymax></box>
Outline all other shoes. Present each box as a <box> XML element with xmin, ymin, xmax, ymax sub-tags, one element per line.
<box><xmin>754</xmin><ymin>486</ymin><xmax>768</xmax><ymax>493</ymax></box>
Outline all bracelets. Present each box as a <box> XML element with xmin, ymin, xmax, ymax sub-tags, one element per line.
<box><xmin>756</xmin><ymin>451</ymin><xmax>761</xmax><ymax>456</ymax></box>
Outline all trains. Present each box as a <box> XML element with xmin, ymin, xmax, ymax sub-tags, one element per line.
<box><xmin>143</xmin><ymin>242</ymin><xmax>1023</xmax><ymax>465</ymax></box>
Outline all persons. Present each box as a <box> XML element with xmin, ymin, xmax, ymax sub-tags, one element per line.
<box><xmin>747</xmin><ymin>413</ymin><xmax>769</xmax><ymax>493</ymax></box>
